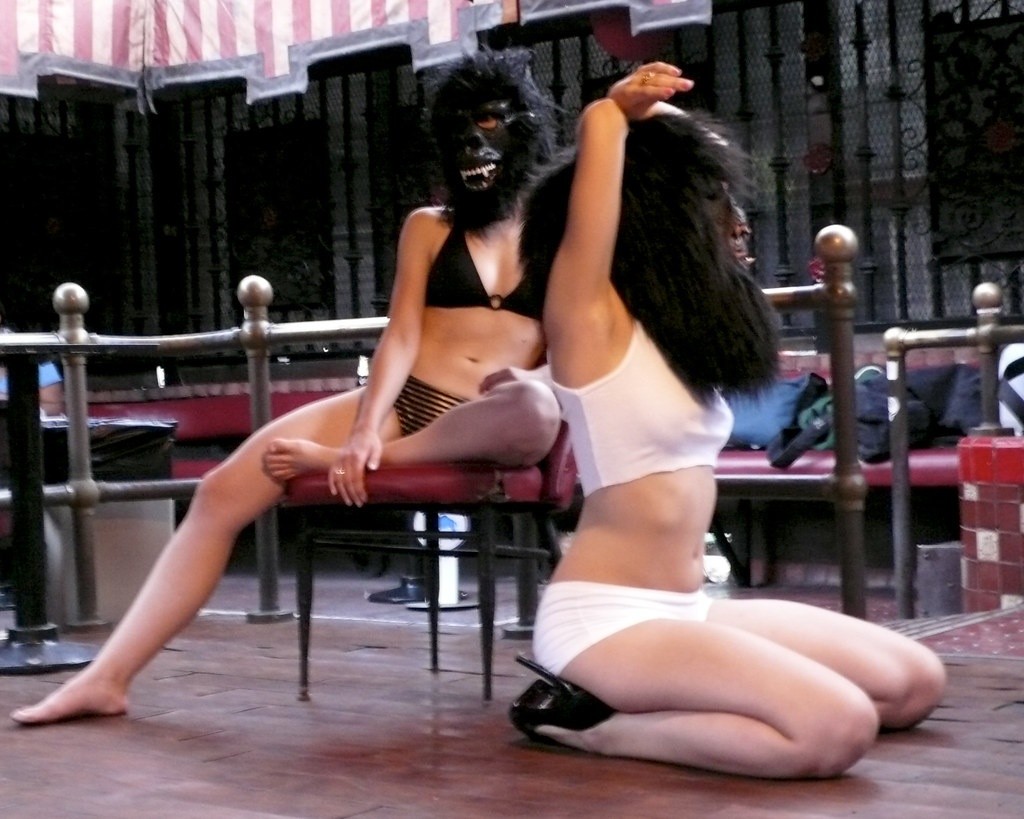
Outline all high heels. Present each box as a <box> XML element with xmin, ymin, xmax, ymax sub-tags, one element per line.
<box><xmin>509</xmin><ymin>653</ymin><xmax>614</xmax><ymax>746</ymax></box>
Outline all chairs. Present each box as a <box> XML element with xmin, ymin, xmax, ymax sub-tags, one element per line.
<box><xmin>291</xmin><ymin>418</ymin><xmax>574</xmax><ymax>706</ymax></box>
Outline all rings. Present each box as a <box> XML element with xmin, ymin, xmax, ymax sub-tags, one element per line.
<box><xmin>333</xmin><ymin>464</ymin><xmax>345</xmax><ymax>476</ymax></box>
<box><xmin>640</xmin><ymin>70</ymin><xmax>654</xmax><ymax>86</ymax></box>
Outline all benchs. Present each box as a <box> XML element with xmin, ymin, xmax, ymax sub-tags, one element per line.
<box><xmin>62</xmin><ymin>346</ymin><xmax>978</xmax><ymax>588</ymax></box>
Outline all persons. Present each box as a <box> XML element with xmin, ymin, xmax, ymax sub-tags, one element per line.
<box><xmin>0</xmin><ymin>329</ymin><xmax>62</xmax><ymax>419</ymax></box>
<box><xmin>511</xmin><ymin>61</ymin><xmax>946</xmax><ymax>779</ymax></box>
<box><xmin>10</xmin><ymin>43</ymin><xmax>564</xmax><ymax>727</ymax></box>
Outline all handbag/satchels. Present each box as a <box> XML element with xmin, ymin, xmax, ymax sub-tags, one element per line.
<box><xmin>763</xmin><ymin>364</ymin><xmax>894</xmax><ymax>469</ymax></box>
<box><xmin>722</xmin><ymin>369</ymin><xmax>826</xmax><ymax>456</ymax></box>
